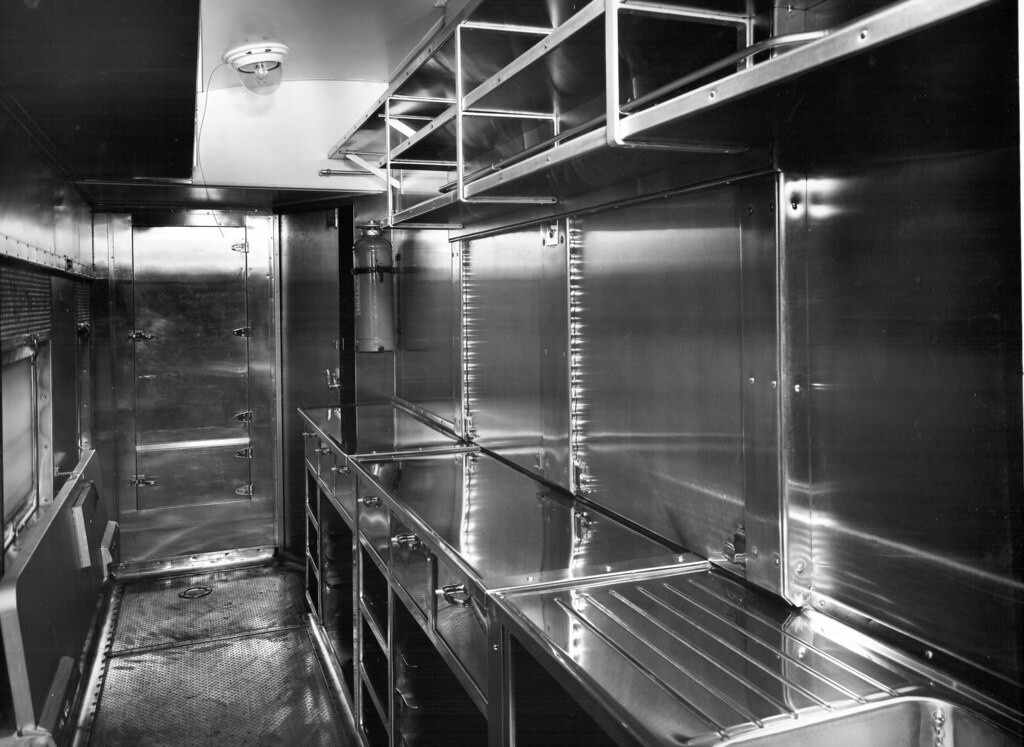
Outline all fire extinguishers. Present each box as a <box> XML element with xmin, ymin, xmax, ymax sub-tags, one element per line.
<box><xmin>353</xmin><ymin>216</ymin><xmax>396</xmax><ymax>355</ymax></box>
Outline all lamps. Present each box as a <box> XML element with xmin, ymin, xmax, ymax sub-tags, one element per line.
<box><xmin>222</xmin><ymin>34</ymin><xmax>292</xmax><ymax>97</ymax></box>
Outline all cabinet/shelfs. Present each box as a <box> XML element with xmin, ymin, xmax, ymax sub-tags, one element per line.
<box><xmin>297</xmin><ymin>403</ymin><xmax>952</xmax><ymax>747</ymax></box>
<box><xmin>327</xmin><ymin>0</ymin><xmax>1019</xmax><ymax>230</ymax></box>
